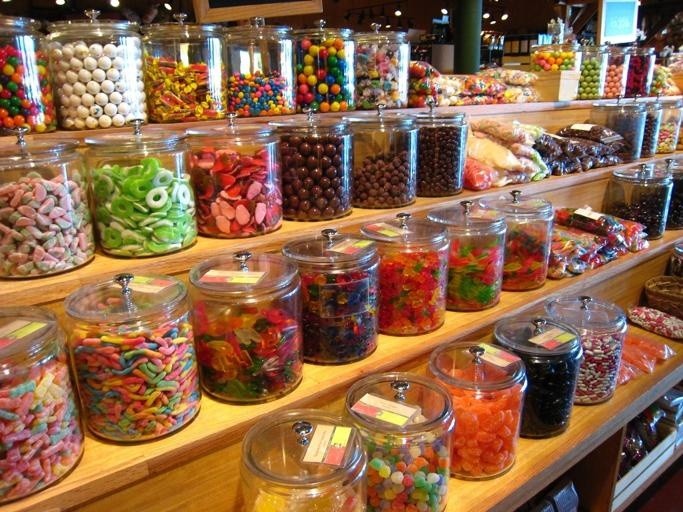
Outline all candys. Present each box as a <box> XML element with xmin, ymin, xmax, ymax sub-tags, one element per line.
<box><xmin>0</xmin><ymin>31</ymin><xmax>683</xmax><ymax>134</ymax></box>
<box><xmin>1</xmin><ymin>109</ymin><xmax>683</xmax><ymax>279</ymax></box>
<box><xmin>0</xmin><ymin>181</ymin><xmax>682</xmax><ymax>511</ymax></box>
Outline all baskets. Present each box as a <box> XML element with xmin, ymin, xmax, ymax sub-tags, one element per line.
<box><xmin>645</xmin><ymin>276</ymin><xmax>683</xmax><ymax>320</ymax></box>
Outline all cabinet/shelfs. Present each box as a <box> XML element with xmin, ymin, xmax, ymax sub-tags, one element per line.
<box><xmin>0</xmin><ymin>93</ymin><xmax>682</xmax><ymax>512</ymax></box>
